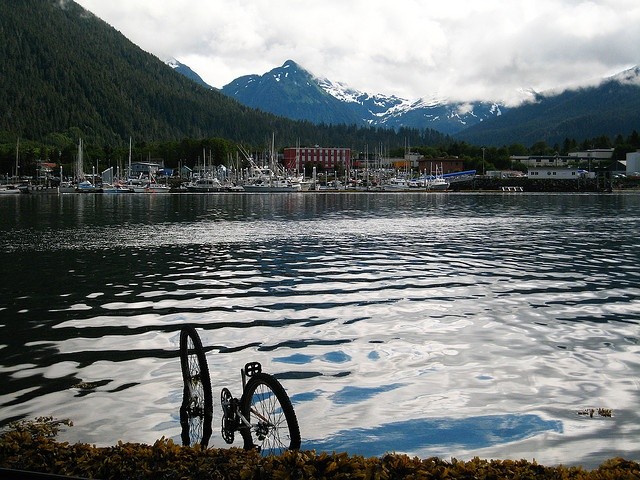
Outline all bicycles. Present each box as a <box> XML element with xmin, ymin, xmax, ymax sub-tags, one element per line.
<box><xmin>180</xmin><ymin>397</ymin><xmax>301</xmax><ymax>455</ymax></box>
<box><xmin>180</xmin><ymin>326</ymin><xmax>300</xmax><ymax>451</ymax></box>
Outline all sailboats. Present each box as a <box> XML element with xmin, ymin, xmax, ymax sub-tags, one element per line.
<box><xmin>184</xmin><ymin>148</ymin><xmax>222</xmax><ymax>191</ymax></box>
<box><xmin>221</xmin><ymin>151</ymin><xmax>245</xmax><ymax>191</ymax></box>
<box><xmin>8</xmin><ymin>136</ymin><xmax>172</xmax><ymax>192</ymax></box>
<box><xmin>244</xmin><ymin>132</ymin><xmax>312</xmax><ymax>191</ymax></box>
<box><xmin>306</xmin><ymin>136</ymin><xmax>450</xmax><ymax>192</ymax></box>
<box><xmin>237</xmin><ymin>144</ymin><xmax>302</xmax><ymax>192</ymax></box>
